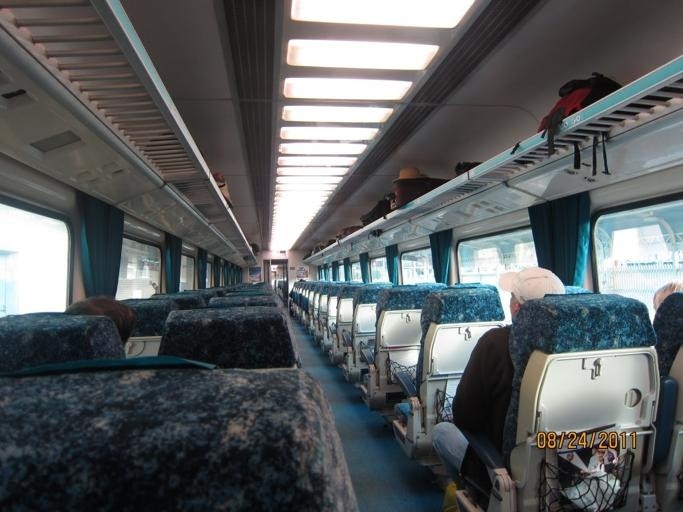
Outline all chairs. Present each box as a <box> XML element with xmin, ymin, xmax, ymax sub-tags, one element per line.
<box><xmin>0</xmin><ymin>277</ymin><xmax>357</xmax><ymax>512</ymax></box>
<box><xmin>288</xmin><ymin>278</ymin><xmax>682</xmax><ymax>512</ymax></box>
<box><xmin>277</xmin><ymin>281</ymin><xmax>287</xmax><ymax>299</ymax></box>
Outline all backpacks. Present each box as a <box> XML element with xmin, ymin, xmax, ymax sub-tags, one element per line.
<box><xmin>536</xmin><ymin>73</ymin><xmax>624</xmax><ymax>133</ymax></box>
<box><xmin>302</xmin><ymin>200</ymin><xmax>389</xmax><ymax>261</ymax></box>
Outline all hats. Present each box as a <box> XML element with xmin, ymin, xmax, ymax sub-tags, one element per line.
<box><xmin>499</xmin><ymin>267</ymin><xmax>567</xmax><ymax>307</ymax></box>
<box><xmin>392</xmin><ymin>165</ymin><xmax>427</xmax><ymax>181</ymax></box>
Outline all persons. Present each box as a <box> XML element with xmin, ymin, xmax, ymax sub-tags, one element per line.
<box><xmin>66</xmin><ymin>297</ymin><xmax>139</xmax><ymax>349</ymax></box>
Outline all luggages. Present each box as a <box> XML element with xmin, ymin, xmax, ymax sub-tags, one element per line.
<box><xmin>389</xmin><ymin>179</ymin><xmax>451</xmax><ymax>211</ymax></box>
